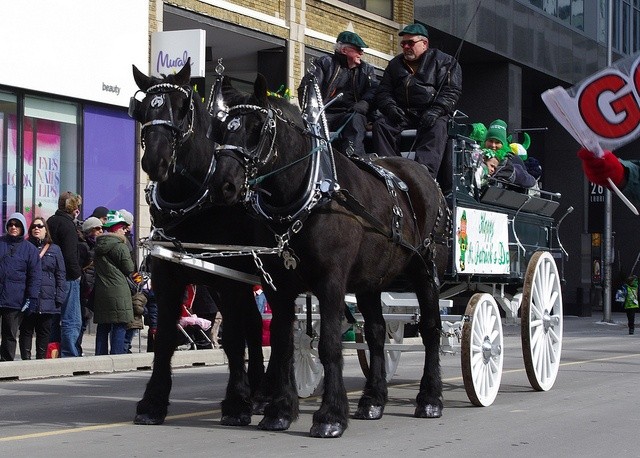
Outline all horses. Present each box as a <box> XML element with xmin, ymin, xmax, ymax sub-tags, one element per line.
<box><xmin>208</xmin><ymin>76</ymin><xmax>449</xmax><ymax>435</ymax></box>
<box><xmin>131</xmin><ymin>56</ymin><xmax>298</xmax><ymax>425</ymax></box>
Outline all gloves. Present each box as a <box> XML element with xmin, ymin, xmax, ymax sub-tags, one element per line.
<box><xmin>384</xmin><ymin>103</ymin><xmax>406</xmax><ymax>125</ymax></box>
<box><xmin>347</xmin><ymin>100</ymin><xmax>370</xmax><ymax>113</ymax></box>
<box><xmin>421</xmin><ymin>106</ymin><xmax>443</xmax><ymax>129</ymax></box>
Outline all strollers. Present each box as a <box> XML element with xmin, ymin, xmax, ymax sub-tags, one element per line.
<box><xmin>131</xmin><ymin>273</ymin><xmax>216</xmax><ymax>350</ymax></box>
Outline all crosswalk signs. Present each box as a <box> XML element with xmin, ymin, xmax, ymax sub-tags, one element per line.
<box><xmin>588</xmin><ymin>180</ymin><xmax>608</xmax><ymax>234</ymax></box>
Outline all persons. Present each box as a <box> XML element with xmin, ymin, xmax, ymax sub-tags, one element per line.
<box><xmin>481</xmin><ymin>119</ymin><xmax>535</xmax><ymax>194</ymax></box>
<box><xmin>46</xmin><ymin>191</ymin><xmax>83</xmax><ymax>358</ymax></box>
<box><xmin>19</xmin><ymin>216</ymin><xmax>66</xmax><ymax>360</ymax></box>
<box><xmin>119</xmin><ymin>208</ymin><xmax>138</xmax><ymax>272</ymax></box>
<box><xmin>620</xmin><ymin>274</ymin><xmax>639</xmax><ymax>334</ymax></box>
<box><xmin>373</xmin><ymin>23</ymin><xmax>463</xmax><ymax>180</ymax></box>
<box><xmin>90</xmin><ymin>207</ymin><xmax>109</xmax><ymax>224</ymax></box>
<box><xmin>123</xmin><ymin>272</ymin><xmax>152</xmax><ymax>354</ymax></box>
<box><xmin>75</xmin><ymin>216</ymin><xmax>103</xmax><ymax>357</ymax></box>
<box><xmin>145</xmin><ymin>284</ymin><xmax>218</xmax><ymax>352</ymax></box>
<box><xmin>470</xmin><ymin>149</ymin><xmax>499</xmax><ymax>189</ymax></box>
<box><xmin>577</xmin><ymin>147</ymin><xmax>640</xmax><ymax>212</ymax></box>
<box><xmin>297</xmin><ymin>31</ymin><xmax>377</xmax><ymax>156</ymax></box>
<box><xmin>0</xmin><ymin>212</ymin><xmax>42</xmax><ymax>361</ymax></box>
<box><xmin>93</xmin><ymin>210</ymin><xmax>135</xmax><ymax>355</ymax></box>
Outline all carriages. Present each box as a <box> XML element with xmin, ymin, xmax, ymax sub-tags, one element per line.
<box><xmin>128</xmin><ymin>57</ymin><xmax>573</xmax><ymax>438</ymax></box>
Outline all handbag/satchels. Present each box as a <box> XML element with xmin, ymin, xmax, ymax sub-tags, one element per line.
<box><xmin>104</xmin><ymin>251</ymin><xmax>138</xmax><ymax>296</ymax></box>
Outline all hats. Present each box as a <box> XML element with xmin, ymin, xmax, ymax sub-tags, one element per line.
<box><xmin>102</xmin><ymin>210</ymin><xmax>130</xmax><ymax>228</ymax></box>
<box><xmin>506</xmin><ymin>132</ymin><xmax>531</xmax><ymax>160</ymax></box>
<box><xmin>58</xmin><ymin>192</ymin><xmax>82</xmax><ymax>213</ymax></box>
<box><xmin>119</xmin><ymin>209</ymin><xmax>134</xmax><ymax>224</ymax></box>
<box><xmin>462</xmin><ymin>122</ymin><xmax>487</xmax><ymax>142</ymax></box>
<box><xmin>336</xmin><ymin>31</ymin><xmax>369</xmax><ymax>48</ymax></box>
<box><xmin>82</xmin><ymin>216</ymin><xmax>103</xmax><ymax>231</ymax></box>
<box><xmin>398</xmin><ymin>24</ymin><xmax>429</xmax><ymax>38</ymax></box>
<box><xmin>488</xmin><ymin>119</ymin><xmax>508</xmax><ymax>144</ymax></box>
<box><xmin>483</xmin><ymin>148</ymin><xmax>500</xmax><ymax>163</ymax></box>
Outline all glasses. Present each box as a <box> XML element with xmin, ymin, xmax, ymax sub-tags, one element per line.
<box><xmin>8</xmin><ymin>222</ymin><xmax>24</xmax><ymax>227</ymax></box>
<box><xmin>400</xmin><ymin>40</ymin><xmax>423</xmax><ymax>47</ymax></box>
<box><xmin>93</xmin><ymin>227</ymin><xmax>103</xmax><ymax>230</ymax></box>
<box><xmin>31</xmin><ymin>224</ymin><xmax>45</xmax><ymax>229</ymax></box>
